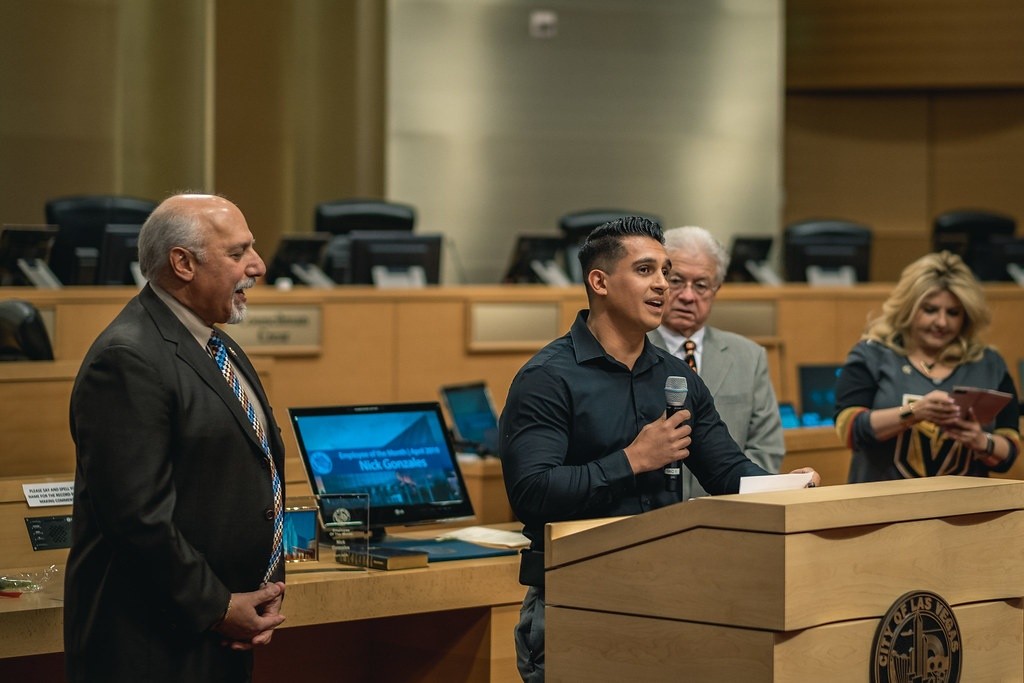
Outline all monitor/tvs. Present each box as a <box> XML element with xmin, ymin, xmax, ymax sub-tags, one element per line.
<box><xmin>442</xmin><ymin>381</ymin><xmax>500</xmax><ymax>457</ymax></box>
<box><xmin>347</xmin><ymin>230</ymin><xmax>441</xmax><ymax>286</ymax></box>
<box><xmin>796</xmin><ymin>244</ymin><xmax>860</xmax><ymax>286</ymax></box>
<box><xmin>798</xmin><ymin>364</ymin><xmax>843</xmax><ymax>426</ymax></box>
<box><xmin>95</xmin><ymin>227</ymin><xmax>148</xmax><ymax>288</ymax></box>
<box><xmin>285</xmin><ymin>400</ymin><xmax>476</xmax><ymax>547</ymax></box>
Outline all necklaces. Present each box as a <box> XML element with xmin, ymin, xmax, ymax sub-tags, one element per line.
<box><xmin>913</xmin><ymin>350</ymin><xmax>938</xmax><ymax>377</ymax></box>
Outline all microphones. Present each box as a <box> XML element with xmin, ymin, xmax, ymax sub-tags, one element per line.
<box><xmin>664</xmin><ymin>376</ymin><xmax>688</xmax><ymax>492</ymax></box>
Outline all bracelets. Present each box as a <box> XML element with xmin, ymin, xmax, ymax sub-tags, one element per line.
<box><xmin>974</xmin><ymin>432</ymin><xmax>995</xmax><ymax>457</ymax></box>
<box><xmin>899</xmin><ymin>402</ymin><xmax>918</xmax><ymax>428</ymax></box>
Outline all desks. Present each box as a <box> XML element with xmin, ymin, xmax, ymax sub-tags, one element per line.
<box><xmin>0</xmin><ymin>284</ymin><xmax>1024</xmax><ymax>683</ymax></box>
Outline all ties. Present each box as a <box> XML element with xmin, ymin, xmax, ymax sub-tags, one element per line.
<box><xmin>682</xmin><ymin>340</ymin><xmax>697</xmax><ymax>373</ymax></box>
<box><xmin>205</xmin><ymin>329</ymin><xmax>284</xmax><ymax>589</ymax></box>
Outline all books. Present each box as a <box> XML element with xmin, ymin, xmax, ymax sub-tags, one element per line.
<box><xmin>335</xmin><ymin>545</ymin><xmax>432</xmax><ymax>571</ymax></box>
<box><xmin>441</xmin><ymin>523</ymin><xmax>533</xmax><ymax>551</ymax></box>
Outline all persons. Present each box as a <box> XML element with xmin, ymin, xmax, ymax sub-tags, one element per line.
<box><xmin>832</xmin><ymin>251</ymin><xmax>1022</xmax><ymax>481</ymax></box>
<box><xmin>498</xmin><ymin>216</ymin><xmax>824</xmax><ymax>683</ymax></box>
<box><xmin>61</xmin><ymin>194</ymin><xmax>288</xmax><ymax>683</ymax></box>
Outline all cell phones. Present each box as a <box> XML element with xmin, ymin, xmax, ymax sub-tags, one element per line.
<box><xmin>948</xmin><ymin>387</ymin><xmax>983</xmax><ymax>423</ymax></box>
